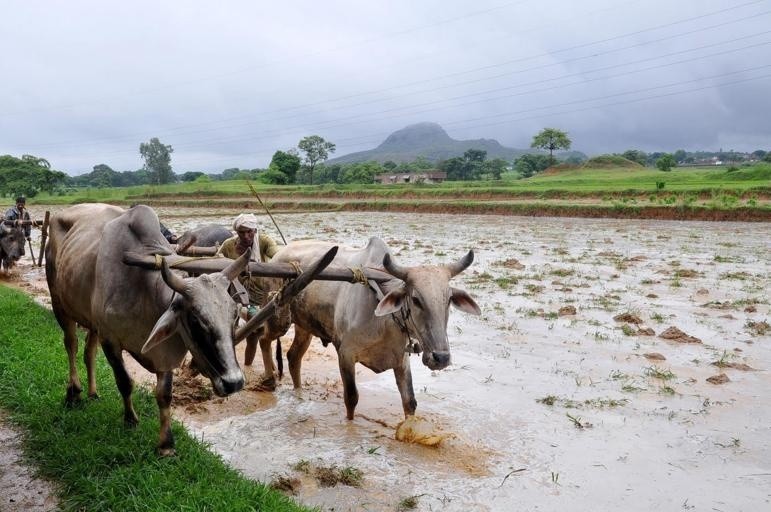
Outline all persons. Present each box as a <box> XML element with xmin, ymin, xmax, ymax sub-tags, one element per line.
<box><xmin>3</xmin><ymin>195</ymin><xmax>31</xmax><ymax>273</ymax></box>
<box><xmin>214</xmin><ymin>210</ymin><xmax>280</xmax><ymax>369</ymax></box>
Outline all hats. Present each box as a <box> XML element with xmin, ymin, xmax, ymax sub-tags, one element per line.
<box><xmin>15</xmin><ymin>196</ymin><xmax>26</xmax><ymax>204</ymax></box>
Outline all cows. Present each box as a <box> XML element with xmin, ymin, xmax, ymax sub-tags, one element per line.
<box><xmin>46</xmin><ymin>202</ymin><xmax>252</xmax><ymax>457</ymax></box>
<box><xmin>0</xmin><ymin>220</ymin><xmax>25</xmax><ymax>273</ymax></box>
<box><xmin>258</xmin><ymin>236</ymin><xmax>482</xmax><ymax>420</ymax></box>
